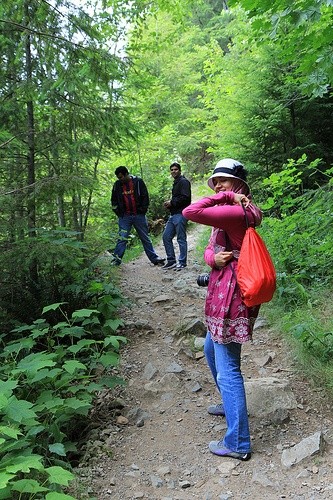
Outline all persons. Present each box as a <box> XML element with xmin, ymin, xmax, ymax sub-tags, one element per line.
<box><xmin>110</xmin><ymin>166</ymin><xmax>166</xmax><ymax>266</ymax></box>
<box><xmin>161</xmin><ymin>162</ymin><xmax>191</xmax><ymax>269</ymax></box>
<box><xmin>182</xmin><ymin>158</ymin><xmax>262</xmax><ymax>461</ymax></box>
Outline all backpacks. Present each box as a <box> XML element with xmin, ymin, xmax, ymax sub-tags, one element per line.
<box><xmin>224</xmin><ymin>201</ymin><xmax>276</xmax><ymax>307</ymax></box>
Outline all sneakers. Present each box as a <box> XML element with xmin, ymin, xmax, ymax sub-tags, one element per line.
<box><xmin>209</xmin><ymin>441</ymin><xmax>251</xmax><ymax>461</ymax></box>
<box><xmin>176</xmin><ymin>264</ymin><xmax>186</xmax><ymax>271</ymax></box>
<box><xmin>207</xmin><ymin>403</ymin><xmax>225</xmax><ymax>415</ymax></box>
<box><xmin>162</xmin><ymin>263</ymin><xmax>176</xmax><ymax>269</ymax></box>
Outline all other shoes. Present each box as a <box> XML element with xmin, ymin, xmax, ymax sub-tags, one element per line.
<box><xmin>111</xmin><ymin>260</ymin><xmax>120</xmax><ymax>266</ymax></box>
<box><xmin>154</xmin><ymin>258</ymin><xmax>165</xmax><ymax>265</ymax></box>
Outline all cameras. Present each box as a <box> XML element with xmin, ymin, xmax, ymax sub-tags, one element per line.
<box><xmin>197</xmin><ymin>275</ymin><xmax>210</xmax><ymax>286</ymax></box>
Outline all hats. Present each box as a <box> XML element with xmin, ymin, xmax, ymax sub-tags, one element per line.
<box><xmin>208</xmin><ymin>159</ymin><xmax>251</xmax><ymax>196</ymax></box>
